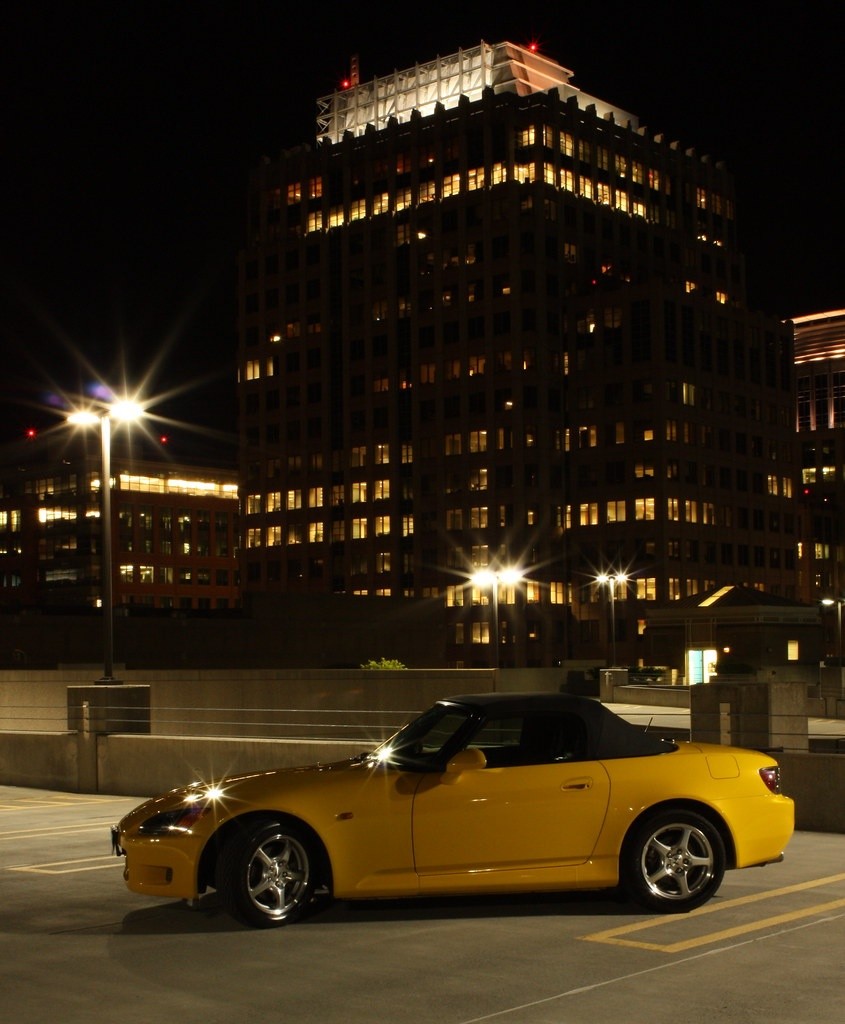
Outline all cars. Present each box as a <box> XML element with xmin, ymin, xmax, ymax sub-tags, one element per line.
<box><xmin>112</xmin><ymin>691</ymin><xmax>797</xmax><ymax>930</ymax></box>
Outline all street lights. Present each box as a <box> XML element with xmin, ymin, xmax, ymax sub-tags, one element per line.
<box><xmin>597</xmin><ymin>569</ymin><xmax>630</xmax><ymax>668</ymax></box>
<box><xmin>472</xmin><ymin>562</ymin><xmax>523</xmax><ymax>696</ymax></box>
<box><xmin>65</xmin><ymin>392</ymin><xmax>147</xmax><ymax>684</ymax></box>
